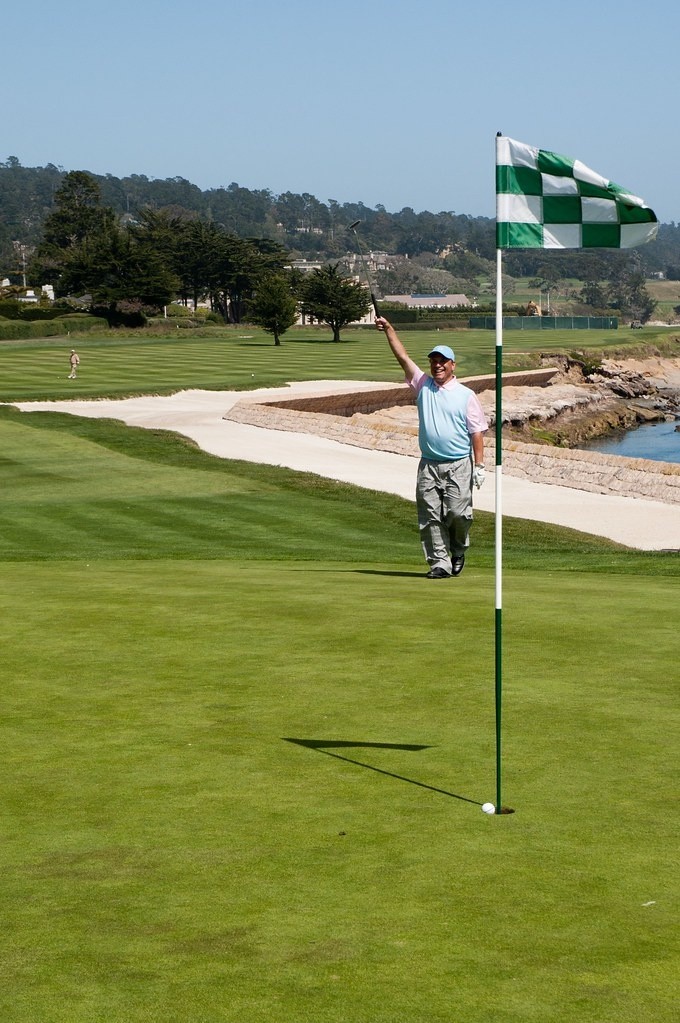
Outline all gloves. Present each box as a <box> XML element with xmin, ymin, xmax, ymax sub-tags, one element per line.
<box><xmin>472</xmin><ymin>462</ymin><xmax>485</xmax><ymax>490</ymax></box>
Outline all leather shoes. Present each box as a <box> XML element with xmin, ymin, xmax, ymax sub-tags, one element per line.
<box><xmin>425</xmin><ymin>567</ymin><xmax>451</xmax><ymax>578</ymax></box>
<box><xmin>451</xmin><ymin>554</ymin><xmax>465</xmax><ymax>575</ymax></box>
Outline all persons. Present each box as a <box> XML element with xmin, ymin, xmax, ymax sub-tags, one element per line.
<box><xmin>68</xmin><ymin>348</ymin><xmax>81</xmax><ymax>380</ymax></box>
<box><xmin>374</xmin><ymin>316</ymin><xmax>489</xmax><ymax>578</ymax></box>
<box><xmin>631</xmin><ymin>321</ymin><xmax>643</xmax><ymax>330</ymax></box>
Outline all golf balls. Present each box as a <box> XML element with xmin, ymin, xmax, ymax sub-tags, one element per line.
<box><xmin>481</xmin><ymin>802</ymin><xmax>496</xmax><ymax>815</ymax></box>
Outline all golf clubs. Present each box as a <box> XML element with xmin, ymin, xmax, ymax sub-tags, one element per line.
<box><xmin>347</xmin><ymin>219</ymin><xmax>385</xmax><ymax>332</ymax></box>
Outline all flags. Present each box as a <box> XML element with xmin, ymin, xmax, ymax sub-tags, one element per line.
<box><xmin>494</xmin><ymin>135</ymin><xmax>659</xmax><ymax>249</ymax></box>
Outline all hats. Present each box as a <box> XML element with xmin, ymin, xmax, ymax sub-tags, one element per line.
<box><xmin>428</xmin><ymin>345</ymin><xmax>455</xmax><ymax>362</ymax></box>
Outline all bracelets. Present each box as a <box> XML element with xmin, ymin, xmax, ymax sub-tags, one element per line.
<box><xmin>475</xmin><ymin>462</ymin><xmax>486</xmax><ymax>468</ymax></box>
